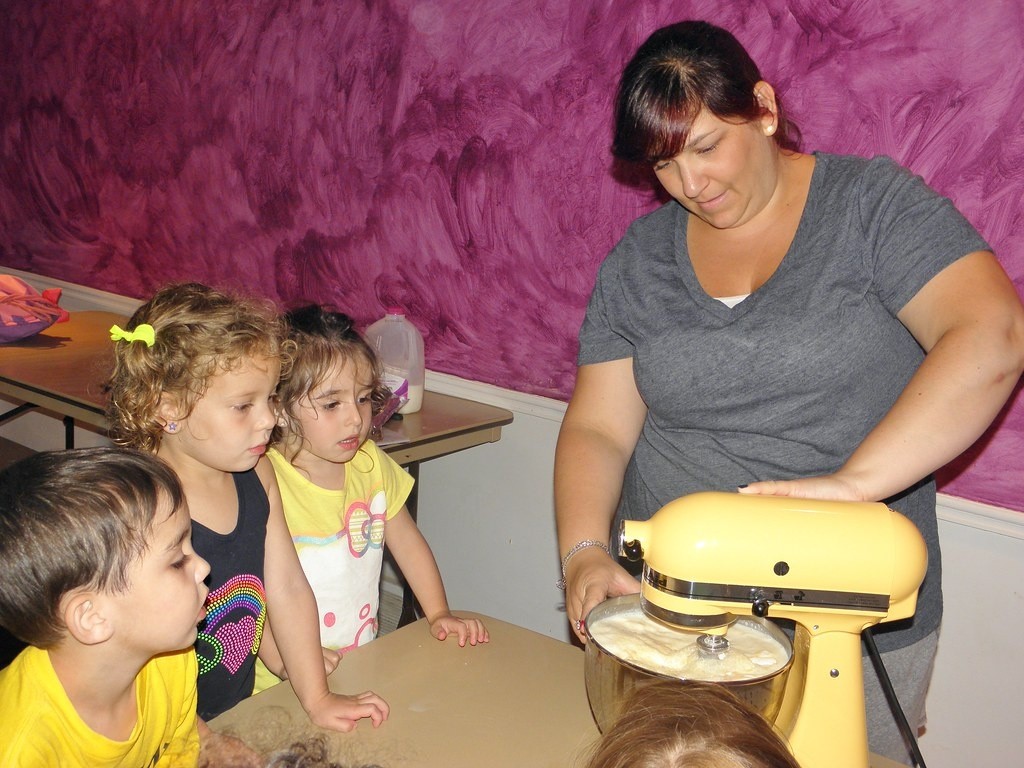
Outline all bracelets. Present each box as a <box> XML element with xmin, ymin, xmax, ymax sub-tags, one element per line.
<box><xmin>556</xmin><ymin>541</ymin><xmax>612</xmax><ymax>590</ymax></box>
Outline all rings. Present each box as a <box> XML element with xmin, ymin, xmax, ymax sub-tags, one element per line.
<box><xmin>576</xmin><ymin>621</ymin><xmax>580</xmax><ymax>629</ymax></box>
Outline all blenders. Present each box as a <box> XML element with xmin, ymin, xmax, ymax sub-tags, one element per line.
<box><xmin>617</xmin><ymin>487</ymin><xmax>929</xmax><ymax>768</ymax></box>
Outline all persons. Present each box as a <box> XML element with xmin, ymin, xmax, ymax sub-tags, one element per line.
<box><xmin>0</xmin><ymin>446</ymin><xmax>211</xmax><ymax>768</ymax></box>
<box><xmin>584</xmin><ymin>680</ymin><xmax>802</xmax><ymax>768</ymax></box>
<box><xmin>98</xmin><ymin>283</ymin><xmax>390</xmax><ymax>768</ymax></box>
<box><xmin>553</xmin><ymin>21</ymin><xmax>1024</xmax><ymax>768</ymax></box>
<box><xmin>250</xmin><ymin>305</ymin><xmax>491</xmax><ymax>695</ymax></box>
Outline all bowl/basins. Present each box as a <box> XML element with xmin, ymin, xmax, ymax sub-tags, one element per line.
<box><xmin>581</xmin><ymin>594</ymin><xmax>794</xmax><ymax>746</ymax></box>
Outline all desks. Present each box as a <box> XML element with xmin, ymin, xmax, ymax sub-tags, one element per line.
<box><xmin>0</xmin><ymin>311</ymin><xmax>512</xmax><ymax>627</ymax></box>
<box><xmin>207</xmin><ymin>611</ymin><xmax>914</xmax><ymax>768</ymax></box>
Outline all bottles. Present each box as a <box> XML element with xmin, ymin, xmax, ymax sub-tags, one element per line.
<box><xmin>362</xmin><ymin>307</ymin><xmax>426</xmax><ymax>415</ymax></box>
